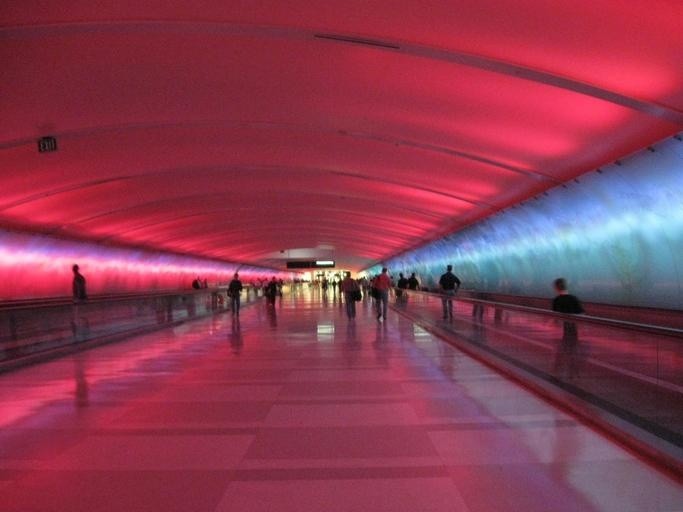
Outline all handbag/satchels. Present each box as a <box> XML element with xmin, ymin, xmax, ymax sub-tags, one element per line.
<box><xmin>352</xmin><ymin>291</ymin><xmax>362</xmax><ymax>300</ymax></box>
<box><xmin>371</xmin><ymin>287</ymin><xmax>382</xmax><ymax>299</ymax></box>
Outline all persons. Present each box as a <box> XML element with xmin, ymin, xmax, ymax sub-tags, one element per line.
<box><xmin>438</xmin><ymin>264</ymin><xmax>461</xmax><ymax>320</ymax></box>
<box><xmin>397</xmin><ymin>272</ymin><xmax>408</xmax><ymax>288</ymax></box>
<box><xmin>552</xmin><ymin>277</ymin><xmax>588</xmax><ymax>370</ymax></box>
<box><xmin>320</xmin><ymin>274</ymin><xmax>394</xmax><ymax>299</ymax></box>
<box><xmin>372</xmin><ymin>267</ymin><xmax>394</xmax><ymax>320</ymax></box>
<box><xmin>191</xmin><ymin>275</ymin><xmax>202</xmax><ymax>290</ymax></box>
<box><xmin>70</xmin><ymin>263</ymin><xmax>89</xmax><ymax>336</ymax></box>
<box><xmin>202</xmin><ymin>278</ymin><xmax>208</xmax><ymax>288</ymax></box>
<box><xmin>228</xmin><ymin>273</ymin><xmax>243</xmax><ymax>316</ymax></box>
<box><xmin>248</xmin><ymin>276</ymin><xmax>285</xmax><ymax>307</ymax></box>
<box><xmin>407</xmin><ymin>272</ymin><xmax>420</xmax><ymax>291</ymax></box>
<box><xmin>340</xmin><ymin>271</ymin><xmax>361</xmax><ymax>321</ymax></box>
